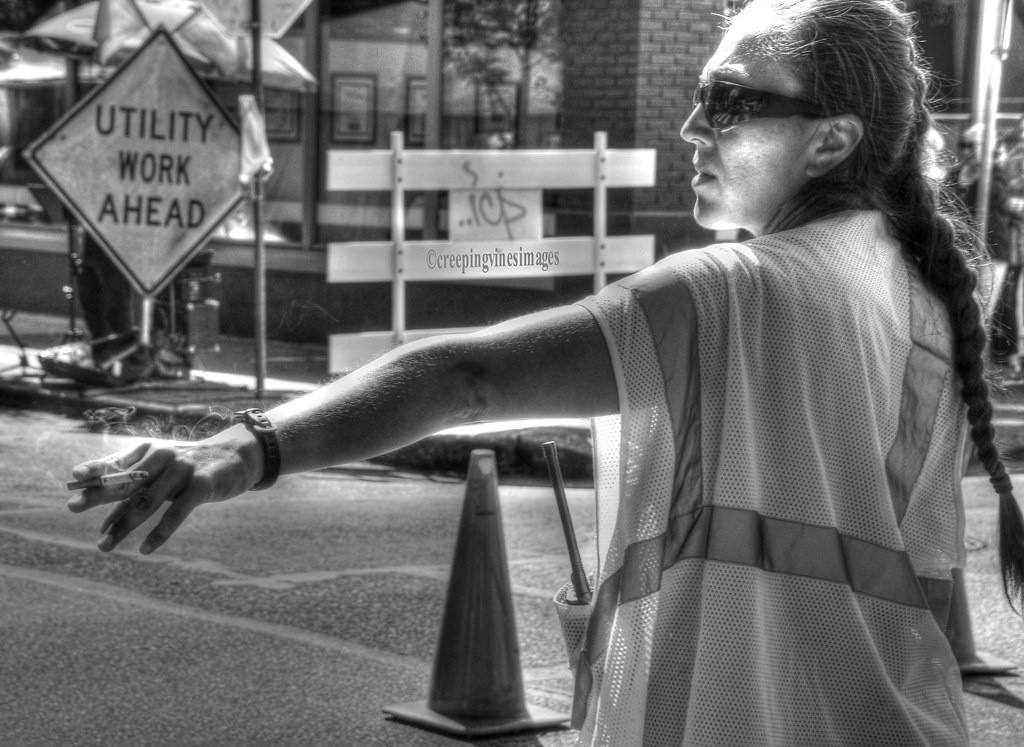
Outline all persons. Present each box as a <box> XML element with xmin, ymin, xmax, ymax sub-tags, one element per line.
<box><xmin>68</xmin><ymin>0</ymin><xmax>1023</xmax><ymax>747</ymax></box>
<box><xmin>937</xmin><ymin>116</ymin><xmax>1024</xmax><ymax>360</ymax></box>
<box><xmin>78</xmin><ymin>229</ymin><xmax>135</xmax><ymax>357</ymax></box>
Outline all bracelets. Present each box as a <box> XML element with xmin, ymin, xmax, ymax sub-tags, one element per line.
<box><xmin>231</xmin><ymin>409</ymin><xmax>280</xmax><ymax>490</ymax></box>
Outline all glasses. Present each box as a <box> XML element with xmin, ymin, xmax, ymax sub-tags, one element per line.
<box><xmin>691</xmin><ymin>82</ymin><xmax>833</xmax><ymax>125</ymax></box>
<box><xmin>958</xmin><ymin>143</ymin><xmax>975</xmax><ymax>149</ymax></box>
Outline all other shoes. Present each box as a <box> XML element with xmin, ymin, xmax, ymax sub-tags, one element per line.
<box><xmin>997</xmin><ymin>364</ymin><xmax>1024</xmax><ymax>386</ymax></box>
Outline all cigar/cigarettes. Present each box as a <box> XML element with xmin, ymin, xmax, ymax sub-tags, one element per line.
<box><xmin>67</xmin><ymin>471</ymin><xmax>149</xmax><ymax>490</ymax></box>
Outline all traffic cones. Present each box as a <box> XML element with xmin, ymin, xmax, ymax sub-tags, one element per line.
<box><xmin>380</xmin><ymin>448</ymin><xmax>571</xmax><ymax>738</ymax></box>
